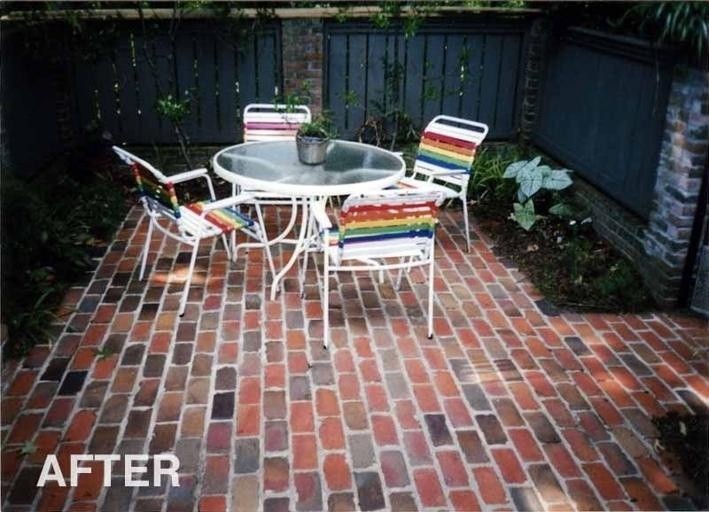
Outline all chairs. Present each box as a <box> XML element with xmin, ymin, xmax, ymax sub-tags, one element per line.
<box><xmin>112</xmin><ymin>102</ymin><xmax>489</xmax><ymax>347</ymax></box>
